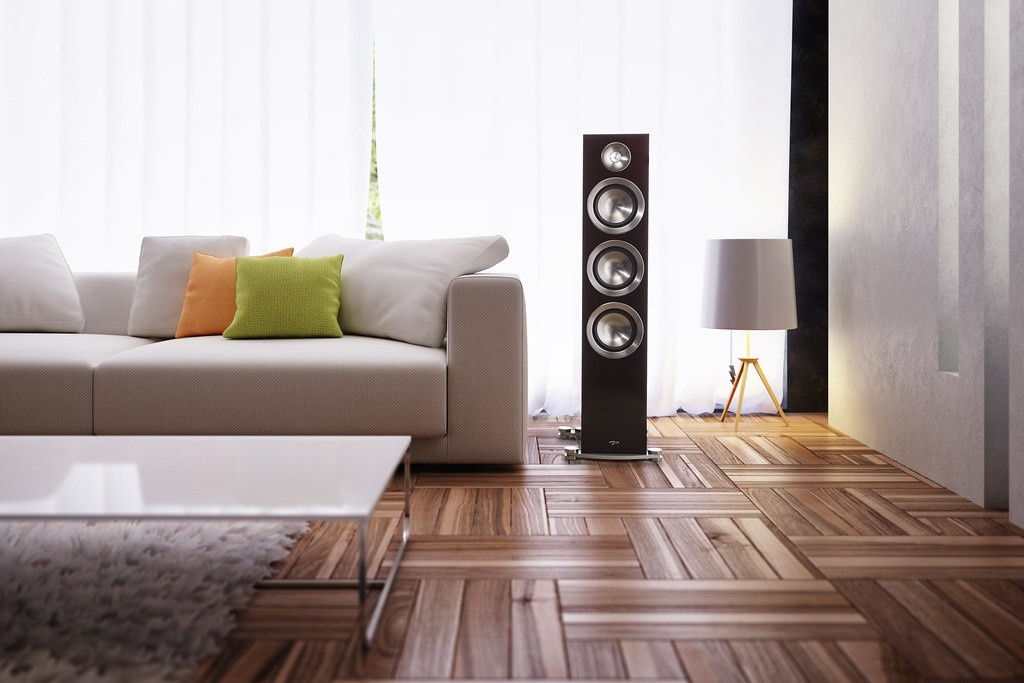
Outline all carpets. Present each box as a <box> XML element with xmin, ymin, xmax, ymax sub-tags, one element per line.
<box><xmin>0</xmin><ymin>518</ymin><xmax>308</xmax><ymax>682</ymax></box>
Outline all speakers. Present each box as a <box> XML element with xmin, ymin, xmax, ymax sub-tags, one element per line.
<box><xmin>584</xmin><ymin>134</ymin><xmax>649</xmax><ymax>456</ymax></box>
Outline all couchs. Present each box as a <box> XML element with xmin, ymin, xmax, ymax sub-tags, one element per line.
<box><xmin>1</xmin><ymin>270</ymin><xmax>533</xmax><ymax>466</ymax></box>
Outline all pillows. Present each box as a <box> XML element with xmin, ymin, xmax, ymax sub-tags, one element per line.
<box><xmin>125</xmin><ymin>233</ymin><xmax>252</xmax><ymax>337</ymax></box>
<box><xmin>173</xmin><ymin>243</ymin><xmax>295</xmax><ymax>337</ymax></box>
<box><xmin>223</xmin><ymin>252</ymin><xmax>349</xmax><ymax>338</ymax></box>
<box><xmin>292</xmin><ymin>234</ymin><xmax>509</xmax><ymax>346</ymax></box>
<box><xmin>2</xmin><ymin>234</ymin><xmax>85</xmax><ymax>334</ymax></box>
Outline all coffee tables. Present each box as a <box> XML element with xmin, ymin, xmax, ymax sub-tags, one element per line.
<box><xmin>2</xmin><ymin>434</ymin><xmax>418</xmax><ymax>653</ymax></box>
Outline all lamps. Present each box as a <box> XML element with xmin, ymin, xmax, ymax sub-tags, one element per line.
<box><xmin>699</xmin><ymin>237</ymin><xmax>798</xmax><ymax>435</ymax></box>
